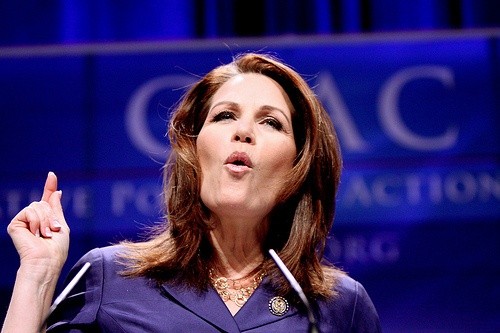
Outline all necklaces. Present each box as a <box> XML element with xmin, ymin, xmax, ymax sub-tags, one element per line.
<box><xmin>199</xmin><ymin>257</ymin><xmax>268</xmax><ymax>306</ymax></box>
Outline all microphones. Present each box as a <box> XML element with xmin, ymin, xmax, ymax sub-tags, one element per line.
<box><xmin>261</xmin><ymin>235</ymin><xmax>321</xmax><ymax>333</ymax></box>
<box><xmin>36</xmin><ymin>260</ymin><xmax>92</xmax><ymax>333</ymax></box>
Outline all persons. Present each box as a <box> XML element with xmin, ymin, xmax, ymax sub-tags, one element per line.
<box><xmin>0</xmin><ymin>55</ymin><xmax>382</xmax><ymax>333</ymax></box>
